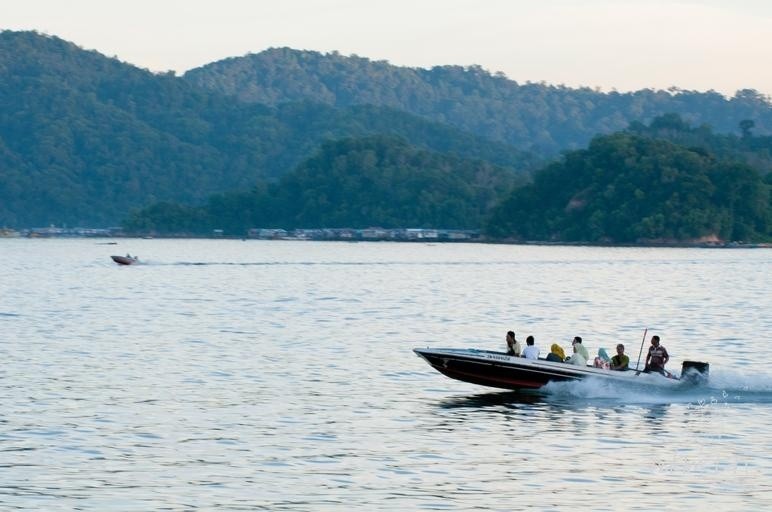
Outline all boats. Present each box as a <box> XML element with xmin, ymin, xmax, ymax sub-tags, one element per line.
<box><xmin>411</xmin><ymin>343</ymin><xmax>714</xmax><ymax>400</ymax></box>
<box><xmin>110</xmin><ymin>254</ymin><xmax>141</xmax><ymax>265</ymax></box>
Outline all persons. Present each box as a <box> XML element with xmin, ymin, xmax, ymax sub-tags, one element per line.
<box><xmin>505</xmin><ymin>330</ymin><xmax>522</xmax><ymax>357</ymax></box>
<box><xmin>609</xmin><ymin>343</ymin><xmax>630</xmax><ymax>370</ymax></box>
<box><xmin>544</xmin><ymin>343</ymin><xmax>566</xmax><ymax>362</ymax></box>
<box><xmin>571</xmin><ymin>336</ymin><xmax>583</xmax><ymax>353</ymax></box>
<box><xmin>565</xmin><ymin>341</ymin><xmax>589</xmax><ymax>366</ymax></box>
<box><xmin>520</xmin><ymin>335</ymin><xmax>541</xmax><ymax>359</ymax></box>
<box><xmin>644</xmin><ymin>334</ymin><xmax>670</xmax><ymax>375</ymax></box>
<box><xmin>590</xmin><ymin>346</ymin><xmax>614</xmax><ymax>370</ymax></box>
